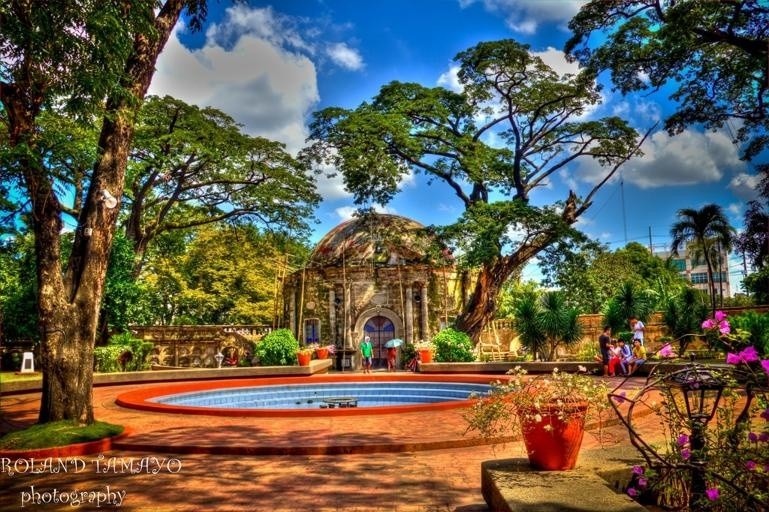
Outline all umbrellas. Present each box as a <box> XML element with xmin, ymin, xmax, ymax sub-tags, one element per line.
<box><xmin>384</xmin><ymin>338</ymin><xmax>404</xmax><ymax>348</ymax></box>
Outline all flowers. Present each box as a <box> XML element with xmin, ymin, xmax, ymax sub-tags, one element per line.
<box><xmin>458</xmin><ymin>363</ymin><xmax>619</xmax><ymax>456</ymax></box>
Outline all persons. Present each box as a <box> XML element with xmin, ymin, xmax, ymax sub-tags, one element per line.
<box><xmin>360</xmin><ymin>336</ymin><xmax>374</xmax><ymax>374</ymax></box>
<box><xmin>599</xmin><ymin>325</ymin><xmax>647</xmax><ymax>378</ymax></box>
<box><xmin>387</xmin><ymin>346</ymin><xmax>396</xmax><ymax>373</ymax></box>
<box><xmin>629</xmin><ymin>316</ymin><xmax>645</xmax><ymax>346</ymax></box>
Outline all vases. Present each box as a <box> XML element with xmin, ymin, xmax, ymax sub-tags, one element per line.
<box><xmin>516</xmin><ymin>405</ymin><xmax>588</xmax><ymax>472</ymax></box>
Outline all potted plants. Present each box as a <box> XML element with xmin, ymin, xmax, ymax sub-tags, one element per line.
<box><xmin>296</xmin><ymin>347</ymin><xmax>312</xmax><ymax>366</ymax></box>
<box><xmin>316</xmin><ymin>348</ymin><xmax>330</xmax><ymax>359</ymax></box>
<box><xmin>413</xmin><ymin>340</ymin><xmax>436</xmax><ymax>363</ymax></box>
